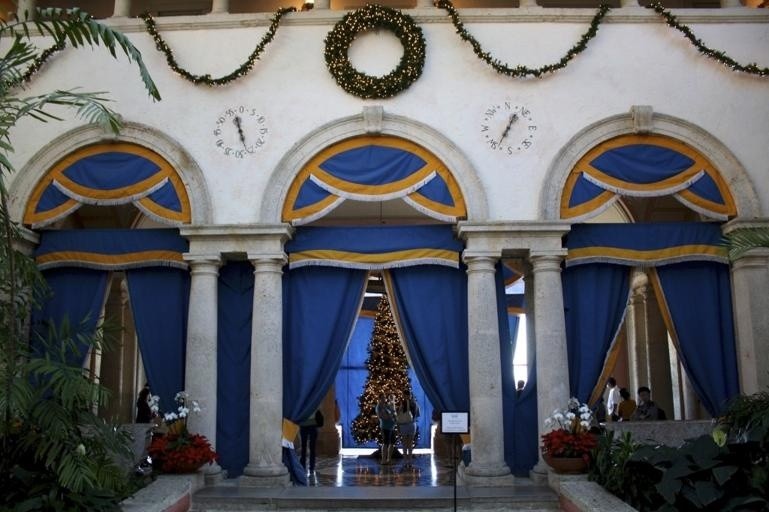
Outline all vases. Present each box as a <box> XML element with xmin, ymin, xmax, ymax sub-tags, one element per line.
<box><xmin>157</xmin><ymin>456</ymin><xmax>210</xmax><ymax>475</ymax></box>
<box><xmin>542</xmin><ymin>453</ymin><xmax>591</xmax><ymax>476</ymax></box>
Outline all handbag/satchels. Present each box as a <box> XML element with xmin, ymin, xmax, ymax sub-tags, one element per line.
<box><xmin>315</xmin><ymin>410</ymin><xmax>324</xmax><ymax>428</ymax></box>
<box><xmin>396</xmin><ymin>411</ymin><xmax>413</xmax><ymax>425</ymax></box>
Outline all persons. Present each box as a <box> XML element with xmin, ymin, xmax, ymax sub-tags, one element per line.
<box><xmin>386</xmin><ymin>393</ymin><xmax>397</xmax><ymax>414</ymax></box>
<box><xmin>605</xmin><ymin>377</ymin><xmax>625</xmax><ymax>422</ymax></box>
<box><xmin>515</xmin><ymin>380</ymin><xmax>525</xmax><ymax>390</ymax></box>
<box><xmin>397</xmin><ymin>389</ymin><xmax>420</xmax><ymax>464</ymax></box>
<box><xmin>135</xmin><ymin>387</ymin><xmax>152</xmax><ymax>423</ymax></box>
<box><xmin>375</xmin><ymin>394</ymin><xmax>396</xmax><ymax>464</ymax></box>
<box><xmin>613</xmin><ymin>389</ymin><xmax>636</xmax><ymax>422</ymax></box>
<box><xmin>630</xmin><ymin>386</ymin><xmax>659</xmax><ymax>422</ymax></box>
<box><xmin>299</xmin><ymin>406</ymin><xmax>324</xmax><ymax>470</ymax></box>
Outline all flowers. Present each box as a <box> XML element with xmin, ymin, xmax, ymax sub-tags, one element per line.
<box><xmin>536</xmin><ymin>391</ymin><xmax>600</xmax><ymax>464</ymax></box>
<box><xmin>139</xmin><ymin>384</ymin><xmax>225</xmax><ymax>475</ymax></box>
